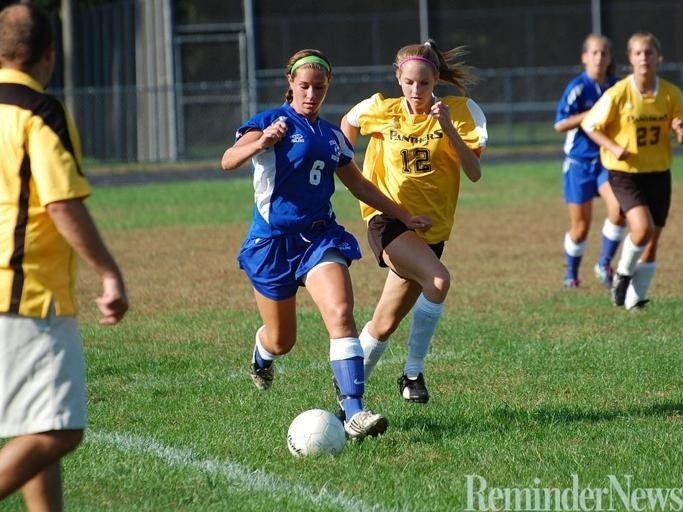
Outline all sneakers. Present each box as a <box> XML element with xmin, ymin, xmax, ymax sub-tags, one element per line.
<box><xmin>399</xmin><ymin>372</ymin><xmax>429</xmax><ymax>405</ymax></box>
<box><xmin>343</xmin><ymin>409</ymin><xmax>387</xmax><ymax>438</ymax></box>
<box><xmin>595</xmin><ymin>262</ymin><xmax>613</xmax><ymax>285</ymax></box>
<box><xmin>611</xmin><ymin>272</ymin><xmax>632</xmax><ymax>306</ymax></box>
<box><xmin>562</xmin><ymin>279</ymin><xmax>580</xmax><ymax>289</ymax></box>
<box><xmin>251</xmin><ymin>346</ymin><xmax>275</xmax><ymax>390</ymax></box>
<box><xmin>331</xmin><ymin>377</ymin><xmax>349</xmax><ymax>420</ymax></box>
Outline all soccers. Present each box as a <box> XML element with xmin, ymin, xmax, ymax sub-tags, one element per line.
<box><xmin>288</xmin><ymin>409</ymin><xmax>346</xmax><ymax>459</ymax></box>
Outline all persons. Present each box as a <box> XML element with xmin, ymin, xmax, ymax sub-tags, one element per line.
<box><xmin>0</xmin><ymin>2</ymin><xmax>129</xmax><ymax>512</ymax></box>
<box><xmin>340</xmin><ymin>39</ymin><xmax>489</xmax><ymax>404</ymax></box>
<box><xmin>578</xmin><ymin>32</ymin><xmax>683</xmax><ymax>310</ymax></box>
<box><xmin>553</xmin><ymin>34</ymin><xmax>625</xmax><ymax>287</ymax></box>
<box><xmin>219</xmin><ymin>50</ymin><xmax>434</xmax><ymax>438</ymax></box>
